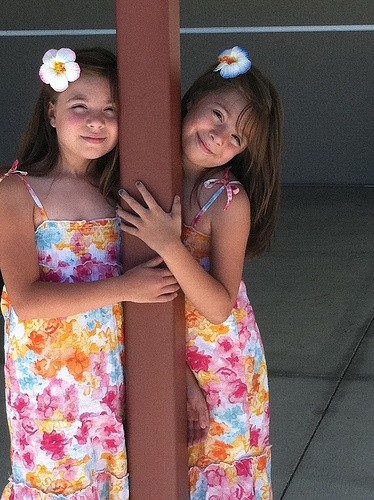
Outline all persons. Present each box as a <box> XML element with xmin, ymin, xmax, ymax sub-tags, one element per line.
<box><xmin>114</xmin><ymin>44</ymin><xmax>285</xmax><ymax>499</ymax></box>
<box><xmin>0</xmin><ymin>46</ymin><xmax>211</xmax><ymax>500</ymax></box>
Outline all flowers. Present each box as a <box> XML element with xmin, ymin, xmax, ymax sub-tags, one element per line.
<box><xmin>39</xmin><ymin>47</ymin><xmax>81</xmax><ymax>92</ymax></box>
<box><xmin>215</xmin><ymin>46</ymin><xmax>252</xmax><ymax>79</ymax></box>
